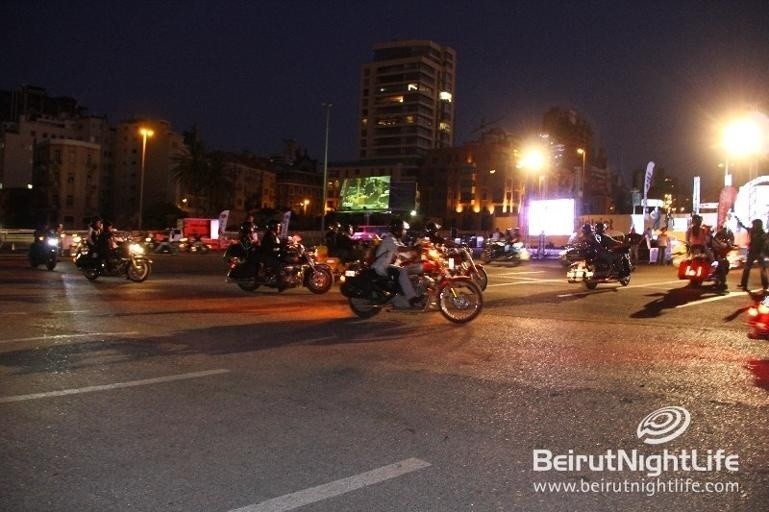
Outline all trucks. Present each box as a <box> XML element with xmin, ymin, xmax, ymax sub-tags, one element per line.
<box><xmin>169</xmin><ymin>217</ymin><xmax>219</xmax><ymax>243</ymax></box>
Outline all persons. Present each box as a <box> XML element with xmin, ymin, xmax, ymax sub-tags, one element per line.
<box><xmin>224</xmin><ymin>212</ymin><xmax>526</xmax><ymax>312</ymax></box>
<box><xmin>32</xmin><ymin>217</ymin><xmax>124</xmax><ymax>270</ymax></box>
<box><xmin>735</xmin><ymin>218</ymin><xmax>769</xmax><ymax>291</ymax></box>
<box><xmin>563</xmin><ymin>214</ymin><xmax>735</xmax><ymax>295</ymax></box>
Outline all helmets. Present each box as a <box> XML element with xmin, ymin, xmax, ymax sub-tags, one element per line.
<box><xmin>342</xmin><ymin>224</ymin><xmax>353</xmax><ymax>232</ymax></box>
<box><xmin>240</xmin><ymin>223</ymin><xmax>257</xmax><ymax>233</ymax></box>
<box><xmin>266</xmin><ymin>218</ymin><xmax>281</xmax><ymax>230</ymax></box>
<box><xmin>691</xmin><ymin>215</ymin><xmax>702</xmax><ymax>224</ymax></box>
<box><xmin>580</xmin><ymin>223</ymin><xmax>595</xmax><ymax>234</ymax></box>
<box><xmin>331</xmin><ymin>221</ymin><xmax>341</xmax><ymax>230</ymax></box>
<box><xmin>595</xmin><ymin>223</ymin><xmax>608</xmax><ymax>234</ymax></box>
<box><xmin>103</xmin><ymin>219</ymin><xmax>113</xmax><ymax>228</ymax></box>
<box><xmin>390</xmin><ymin>218</ymin><xmax>410</xmax><ymax>238</ymax></box>
<box><xmin>425</xmin><ymin>221</ymin><xmax>442</xmax><ymax>234</ymax></box>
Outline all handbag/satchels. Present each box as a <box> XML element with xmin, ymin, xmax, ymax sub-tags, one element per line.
<box><xmin>368</xmin><ymin>246</ymin><xmax>377</xmax><ymax>266</ymax></box>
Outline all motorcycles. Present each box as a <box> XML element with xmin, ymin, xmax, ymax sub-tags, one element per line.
<box><xmin>560</xmin><ymin>237</ymin><xmax>632</xmax><ymax>298</ymax></box>
<box><xmin>223</xmin><ymin>235</ymin><xmax>531</xmax><ymax>324</ymax></box>
<box><xmin>677</xmin><ymin>237</ymin><xmax>739</xmax><ymax>293</ymax></box>
<box><xmin>73</xmin><ymin>238</ymin><xmax>153</xmax><ymax>282</ymax></box>
<box><xmin>27</xmin><ymin>233</ymin><xmax>66</xmax><ymax>271</ymax></box>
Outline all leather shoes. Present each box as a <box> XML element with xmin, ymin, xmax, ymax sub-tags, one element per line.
<box><xmin>410</xmin><ymin>294</ymin><xmax>425</xmax><ymax>306</ymax></box>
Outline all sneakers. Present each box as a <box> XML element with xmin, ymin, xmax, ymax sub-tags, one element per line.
<box><xmin>619</xmin><ymin>271</ymin><xmax>629</xmax><ymax>277</ymax></box>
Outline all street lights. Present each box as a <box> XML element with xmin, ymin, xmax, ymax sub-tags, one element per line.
<box><xmin>576</xmin><ymin>147</ymin><xmax>586</xmax><ymax>209</ymax></box>
<box><xmin>138</xmin><ymin>127</ymin><xmax>154</xmax><ymax>230</ymax></box>
